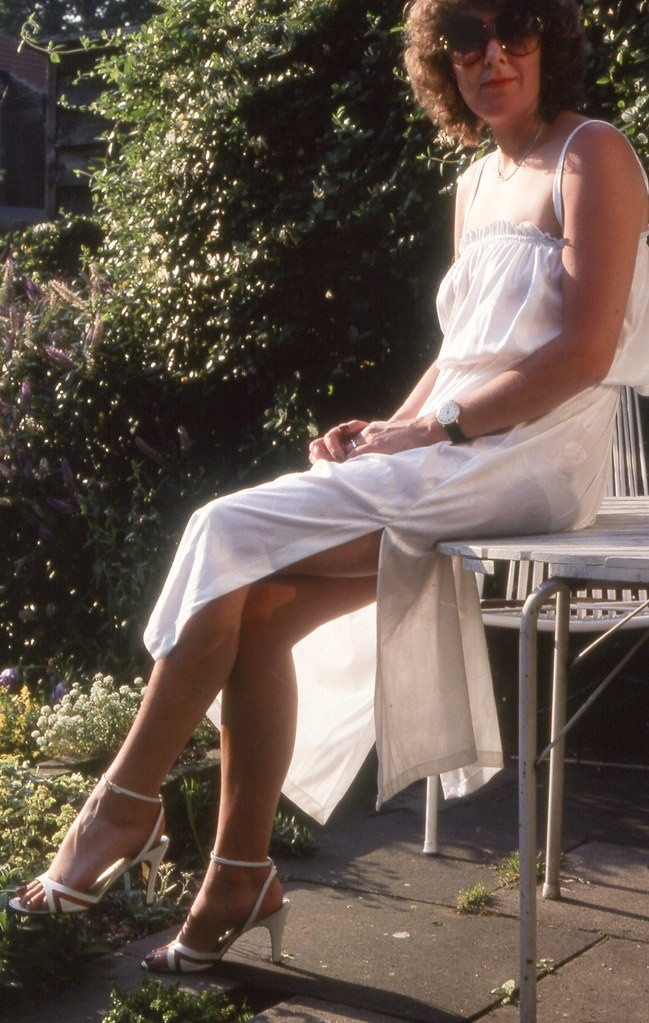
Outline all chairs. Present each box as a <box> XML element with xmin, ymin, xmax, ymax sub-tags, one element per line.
<box><xmin>420</xmin><ymin>387</ymin><xmax>649</xmax><ymax>855</ymax></box>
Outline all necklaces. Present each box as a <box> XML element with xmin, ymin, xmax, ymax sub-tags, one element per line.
<box><xmin>498</xmin><ymin>119</ymin><xmax>545</xmax><ymax>180</ymax></box>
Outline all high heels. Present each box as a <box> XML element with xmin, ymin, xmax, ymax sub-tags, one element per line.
<box><xmin>140</xmin><ymin>850</ymin><xmax>292</xmax><ymax>974</ymax></box>
<box><xmin>7</xmin><ymin>772</ymin><xmax>170</xmax><ymax>915</ymax></box>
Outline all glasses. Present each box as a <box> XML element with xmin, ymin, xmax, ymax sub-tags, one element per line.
<box><xmin>438</xmin><ymin>5</ymin><xmax>546</xmax><ymax>66</ymax></box>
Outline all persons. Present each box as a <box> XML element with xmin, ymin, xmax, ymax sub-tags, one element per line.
<box><xmin>10</xmin><ymin>0</ymin><xmax>649</xmax><ymax>975</ymax></box>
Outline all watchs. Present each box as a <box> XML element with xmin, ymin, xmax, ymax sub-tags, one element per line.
<box><xmin>436</xmin><ymin>398</ymin><xmax>466</xmax><ymax>444</ymax></box>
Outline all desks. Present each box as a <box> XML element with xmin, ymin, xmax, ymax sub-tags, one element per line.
<box><xmin>435</xmin><ymin>494</ymin><xmax>649</xmax><ymax>1023</ymax></box>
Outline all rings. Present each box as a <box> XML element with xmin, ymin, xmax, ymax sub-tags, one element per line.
<box><xmin>350</xmin><ymin>437</ymin><xmax>357</xmax><ymax>448</ymax></box>
